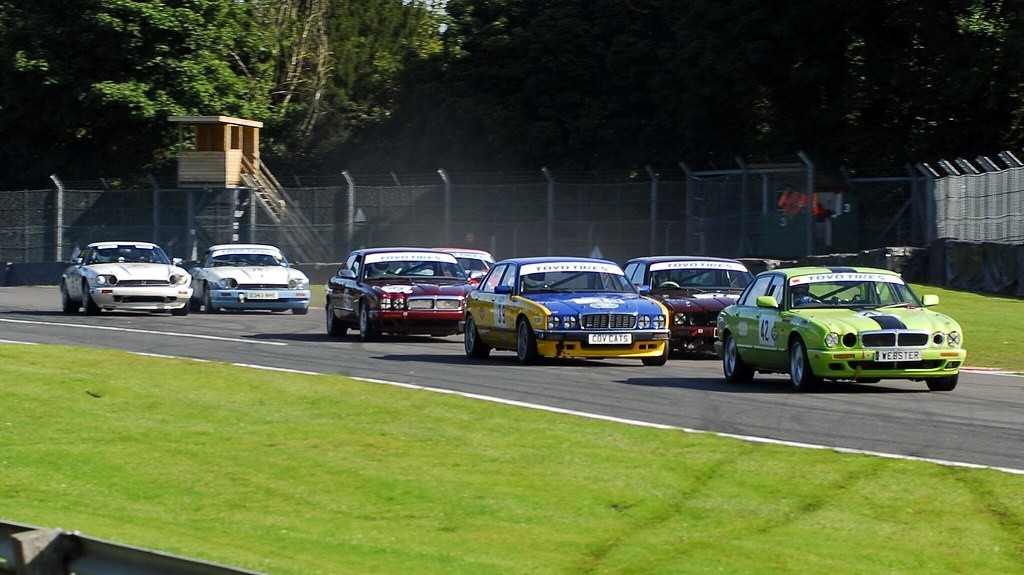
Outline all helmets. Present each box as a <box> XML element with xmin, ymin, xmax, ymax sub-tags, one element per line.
<box><xmin>524</xmin><ymin>273</ymin><xmax>546</xmax><ymax>286</ymax></box>
<box><xmin>792</xmin><ymin>283</ymin><xmax>809</xmax><ymax>300</ymax></box>
<box><xmin>96</xmin><ymin>249</ymin><xmax>111</xmax><ymax>262</ymax></box>
<box><xmin>653</xmin><ymin>271</ymin><xmax>669</xmax><ymax>286</ymax></box>
<box><xmin>372</xmin><ymin>262</ymin><xmax>389</xmax><ymax>274</ymax></box>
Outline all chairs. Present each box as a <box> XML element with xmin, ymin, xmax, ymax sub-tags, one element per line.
<box><xmin>370</xmin><ymin>262</ymin><xmax>391</xmax><ymax>276</ymax></box>
<box><xmin>254</xmin><ymin>257</ymin><xmax>268</xmax><ymax>266</ymax></box>
<box><xmin>132</xmin><ymin>251</ymin><xmax>146</xmax><ymax>263</ymax></box>
<box><xmin>523</xmin><ymin>273</ymin><xmax>546</xmax><ymax>291</ymax></box>
<box><xmin>404</xmin><ymin>261</ymin><xmax>424</xmax><ymax>275</ymax></box>
<box><xmin>653</xmin><ymin>271</ymin><xmax>672</xmax><ymax>287</ymax></box>
<box><xmin>97</xmin><ymin>250</ymin><xmax>112</xmax><ymax>262</ymax></box>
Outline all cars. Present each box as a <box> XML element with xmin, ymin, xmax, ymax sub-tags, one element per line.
<box><xmin>603</xmin><ymin>256</ymin><xmax>758</xmax><ymax>359</ymax></box>
<box><xmin>713</xmin><ymin>265</ymin><xmax>967</xmax><ymax>393</ymax></box>
<box><xmin>190</xmin><ymin>244</ymin><xmax>311</xmax><ymax>316</ymax></box>
<box><xmin>59</xmin><ymin>241</ymin><xmax>194</xmax><ymax>318</ymax></box>
<box><xmin>459</xmin><ymin>256</ymin><xmax>672</xmax><ymax>366</ymax></box>
<box><xmin>325</xmin><ymin>246</ymin><xmax>498</xmax><ymax>343</ymax></box>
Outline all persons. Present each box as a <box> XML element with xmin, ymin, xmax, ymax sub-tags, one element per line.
<box><xmin>654</xmin><ymin>270</ymin><xmax>681</xmax><ymax>288</ymax></box>
<box><xmin>522</xmin><ymin>272</ymin><xmax>555</xmax><ymax>294</ymax></box>
<box><xmin>789</xmin><ymin>283</ymin><xmax>822</xmax><ymax>305</ymax></box>
<box><xmin>367</xmin><ymin>262</ymin><xmax>394</xmax><ymax>276</ymax></box>
